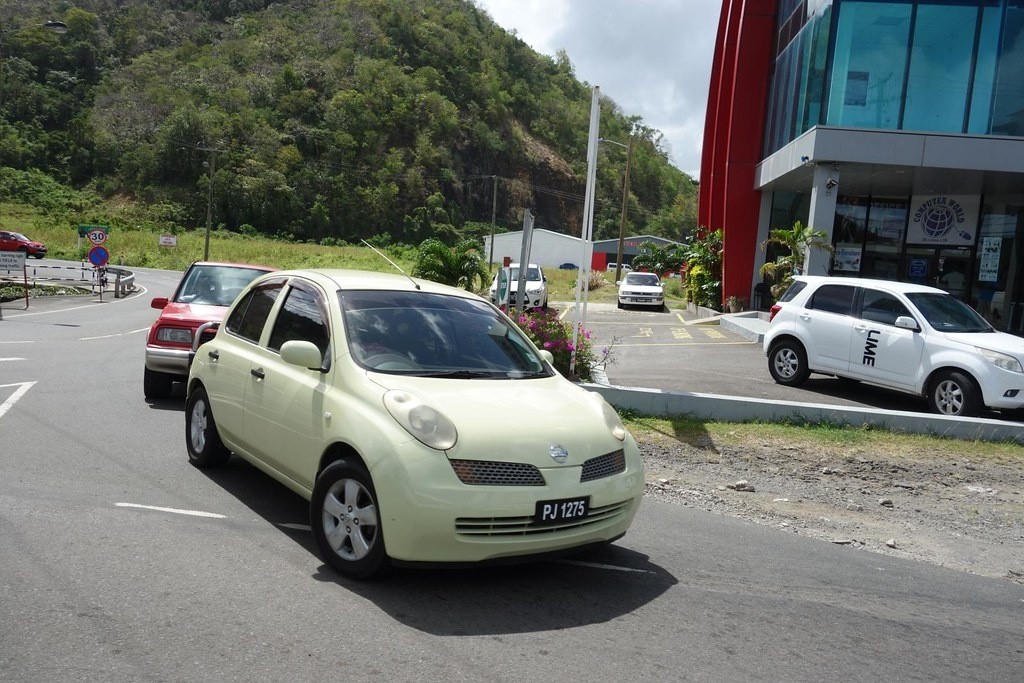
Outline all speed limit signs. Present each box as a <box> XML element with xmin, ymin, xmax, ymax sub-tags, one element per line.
<box><xmin>88</xmin><ymin>228</ymin><xmax>107</xmax><ymax>245</ymax></box>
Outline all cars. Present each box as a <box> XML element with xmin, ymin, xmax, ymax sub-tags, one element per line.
<box><xmin>762</xmin><ymin>275</ymin><xmax>1023</xmax><ymax>418</ymax></box>
<box><xmin>0</xmin><ymin>230</ymin><xmax>48</xmax><ymax>259</ymax></box>
<box><xmin>489</xmin><ymin>262</ymin><xmax>548</xmax><ymax>310</ymax></box>
<box><xmin>144</xmin><ymin>260</ymin><xmax>282</xmax><ymax>401</ymax></box>
<box><xmin>184</xmin><ymin>267</ymin><xmax>645</xmax><ymax>583</ymax></box>
<box><xmin>615</xmin><ymin>271</ymin><xmax>666</xmax><ymax>312</ymax></box>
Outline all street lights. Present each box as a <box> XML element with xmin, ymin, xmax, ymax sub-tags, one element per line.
<box><xmin>597</xmin><ymin>136</ymin><xmax>635</xmax><ymax>281</ymax></box>
<box><xmin>203</xmin><ymin>157</ymin><xmax>216</xmax><ymax>261</ymax></box>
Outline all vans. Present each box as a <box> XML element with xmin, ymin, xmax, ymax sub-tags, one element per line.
<box><xmin>607</xmin><ymin>262</ymin><xmax>635</xmax><ymax>274</ymax></box>
